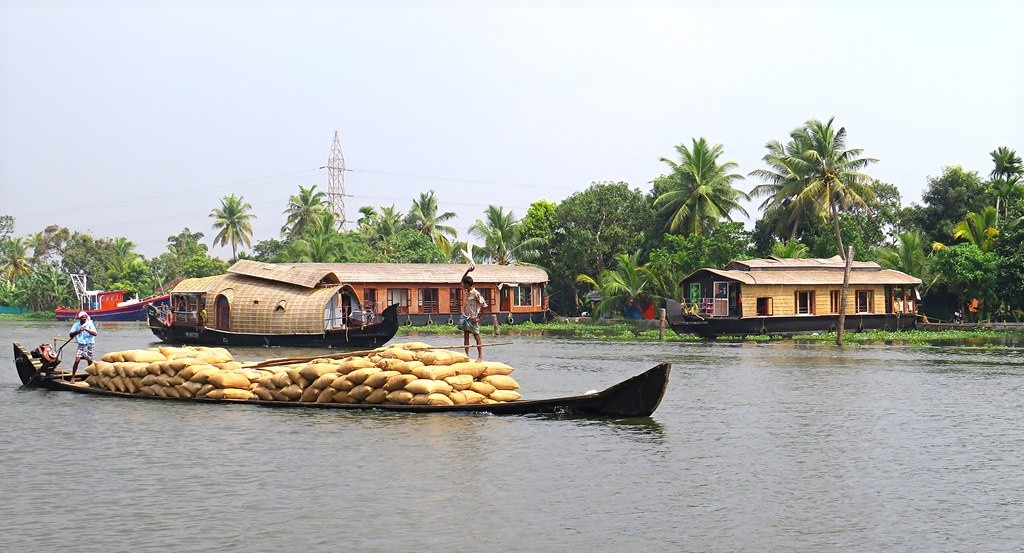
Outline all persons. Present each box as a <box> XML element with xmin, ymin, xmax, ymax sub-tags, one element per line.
<box><xmin>69</xmin><ymin>311</ymin><xmax>97</xmax><ymax>384</ymax></box>
<box><xmin>461</xmin><ymin>263</ymin><xmax>489</xmax><ymax>363</ymax></box>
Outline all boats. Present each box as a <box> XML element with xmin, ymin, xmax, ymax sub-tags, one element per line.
<box><xmin>146</xmin><ymin>259</ymin><xmax>399</xmax><ymax>348</ymax></box>
<box><xmin>54</xmin><ymin>264</ymin><xmax>173</xmax><ymax>321</ymax></box>
<box><xmin>12</xmin><ymin>341</ymin><xmax>672</xmax><ymax>418</ymax></box>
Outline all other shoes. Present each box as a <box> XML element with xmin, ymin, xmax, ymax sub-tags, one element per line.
<box><xmin>70</xmin><ymin>380</ymin><xmax>75</xmax><ymax>384</ymax></box>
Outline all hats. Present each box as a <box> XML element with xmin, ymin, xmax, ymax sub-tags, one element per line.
<box><xmin>78</xmin><ymin>311</ymin><xmax>87</xmax><ymax>318</ymax></box>
